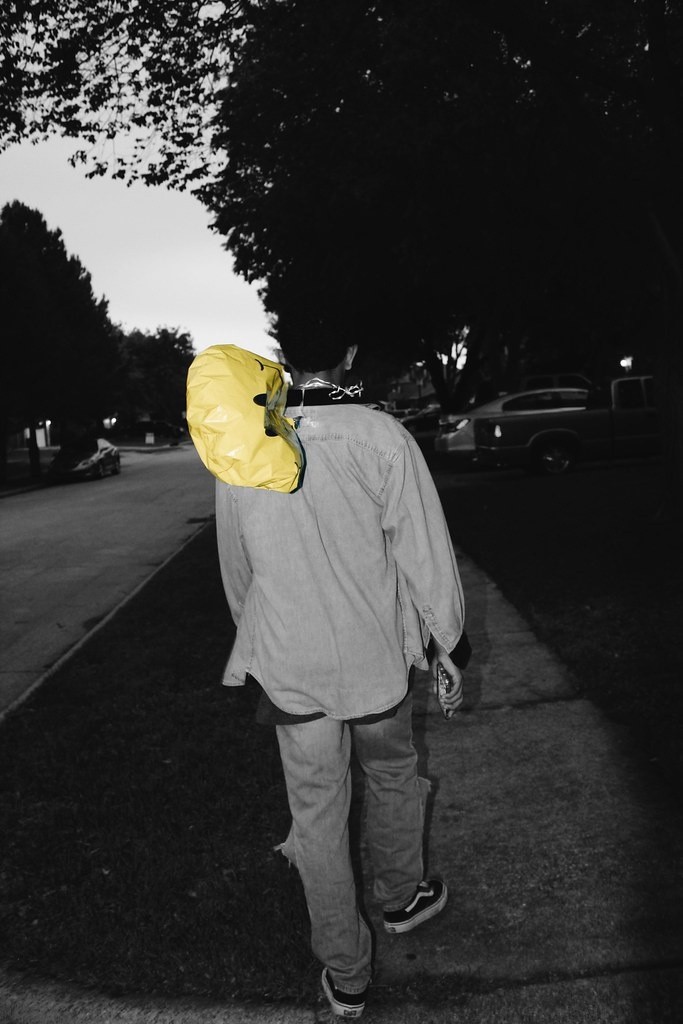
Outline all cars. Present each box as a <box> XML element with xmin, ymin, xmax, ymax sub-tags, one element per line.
<box><xmin>382</xmin><ymin>373</ymin><xmax>599</xmax><ymax>438</ymax></box>
<box><xmin>434</xmin><ymin>387</ymin><xmax>609</xmax><ymax>457</ymax></box>
<box><xmin>49</xmin><ymin>438</ymin><xmax>121</xmax><ymax>482</ymax></box>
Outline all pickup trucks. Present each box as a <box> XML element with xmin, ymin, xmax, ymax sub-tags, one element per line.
<box><xmin>473</xmin><ymin>374</ymin><xmax>665</xmax><ymax>478</ymax></box>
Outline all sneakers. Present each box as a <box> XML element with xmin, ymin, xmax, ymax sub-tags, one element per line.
<box><xmin>382</xmin><ymin>879</ymin><xmax>449</xmax><ymax>933</ymax></box>
<box><xmin>321</xmin><ymin>966</ymin><xmax>373</xmax><ymax>1018</ymax></box>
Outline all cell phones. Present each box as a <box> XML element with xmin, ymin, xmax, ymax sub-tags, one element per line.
<box><xmin>436</xmin><ymin>661</ymin><xmax>452</xmax><ymax>719</ymax></box>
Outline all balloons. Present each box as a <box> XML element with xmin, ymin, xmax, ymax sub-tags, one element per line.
<box><xmin>186</xmin><ymin>344</ymin><xmax>303</xmax><ymax>493</ymax></box>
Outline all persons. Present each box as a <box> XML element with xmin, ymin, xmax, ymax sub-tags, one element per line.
<box><xmin>215</xmin><ymin>299</ymin><xmax>465</xmax><ymax>1018</ymax></box>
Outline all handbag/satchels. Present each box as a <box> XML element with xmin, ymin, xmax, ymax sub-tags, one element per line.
<box><xmin>185</xmin><ymin>343</ymin><xmax>308</xmax><ymax>494</ymax></box>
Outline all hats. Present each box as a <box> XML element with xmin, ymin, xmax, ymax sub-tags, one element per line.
<box><xmin>274</xmin><ymin>301</ymin><xmax>350</xmax><ymax>369</ymax></box>
